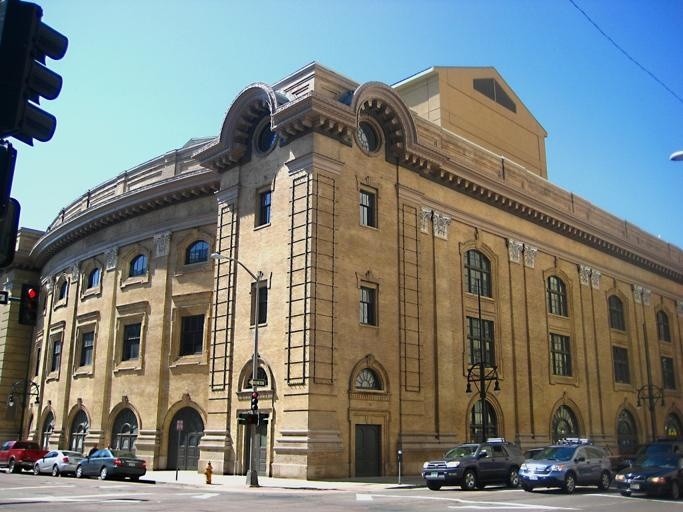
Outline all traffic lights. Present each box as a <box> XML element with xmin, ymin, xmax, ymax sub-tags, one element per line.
<box><xmin>15</xmin><ymin>282</ymin><xmax>40</xmax><ymax>328</ymax></box>
<box><xmin>250</xmin><ymin>391</ymin><xmax>259</xmax><ymax>412</ymax></box>
<box><xmin>0</xmin><ymin>0</ymin><xmax>69</xmax><ymax>147</ymax></box>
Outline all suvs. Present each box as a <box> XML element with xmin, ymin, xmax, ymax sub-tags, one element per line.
<box><xmin>419</xmin><ymin>439</ymin><xmax>527</xmax><ymax>492</ymax></box>
<box><xmin>517</xmin><ymin>441</ymin><xmax>614</xmax><ymax>492</ymax></box>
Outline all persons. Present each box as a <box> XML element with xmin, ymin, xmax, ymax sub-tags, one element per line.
<box><xmin>104</xmin><ymin>443</ymin><xmax>113</xmax><ymax>450</ymax></box>
<box><xmin>88</xmin><ymin>443</ymin><xmax>98</xmax><ymax>456</ymax></box>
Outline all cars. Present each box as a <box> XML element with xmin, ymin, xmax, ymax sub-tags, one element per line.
<box><xmin>33</xmin><ymin>450</ymin><xmax>85</xmax><ymax>477</ymax></box>
<box><xmin>75</xmin><ymin>448</ymin><xmax>146</xmax><ymax>481</ymax></box>
<box><xmin>615</xmin><ymin>440</ymin><xmax>683</xmax><ymax>499</ymax></box>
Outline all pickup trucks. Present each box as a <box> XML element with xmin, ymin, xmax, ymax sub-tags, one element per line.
<box><xmin>0</xmin><ymin>440</ymin><xmax>49</xmax><ymax>473</ymax></box>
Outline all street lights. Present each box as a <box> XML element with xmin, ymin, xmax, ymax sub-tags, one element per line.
<box><xmin>211</xmin><ymin>253</ymin><xmax>263</xmax><ymax>490</ymax></box>
